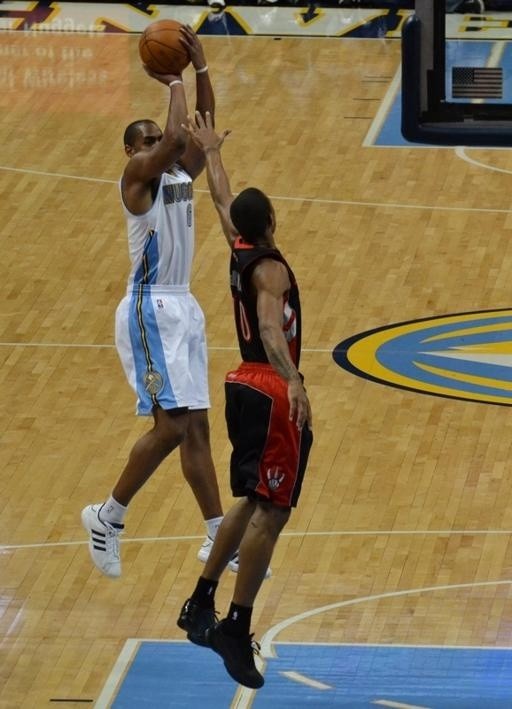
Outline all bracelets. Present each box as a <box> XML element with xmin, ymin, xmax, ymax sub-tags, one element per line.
<box><xmin>169</xmin><ymin>80</ymin><xmax>182</xmax><ymax>87</ymax></box>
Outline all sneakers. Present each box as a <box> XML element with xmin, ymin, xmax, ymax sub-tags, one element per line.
<box><xmin>205</xmin><ymin>618</ymin><xmax>265</xmax><ymax>689</ymax></box>
<box><xmin>197</xmin><ymin>534</ymin><xmax>272</xmax><ymax>580</ymax></box>
<box><xmin>81</xmin><ymin>502</ymin><xmax>124</xmax><ymax>579</ymax></box>
<box><xmin>177</xmin><ymin>598</ymin><xmax>221</xmax><ymax>648</ymax></box>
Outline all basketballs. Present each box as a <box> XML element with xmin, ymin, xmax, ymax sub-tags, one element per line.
<box><xmin>138</xmin><ymin>20</ymin><xmax>191</xmax><ymax>74</ymax></box>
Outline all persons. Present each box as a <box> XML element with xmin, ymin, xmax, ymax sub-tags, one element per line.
<box><xmin>178</xmin><ymin>110</ymin><xmax>311</xmax><ymax>688</ymax></box>
<box><xmin>79</xmin><ymin>23</ymin><xmax>270</xmax><ymax>578</ymax></box>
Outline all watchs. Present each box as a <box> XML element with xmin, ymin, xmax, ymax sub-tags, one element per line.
<box><xmin>195</xmin><ymin>66</ymin><xmax>208</xmax><ymax>73</ymax></box>
